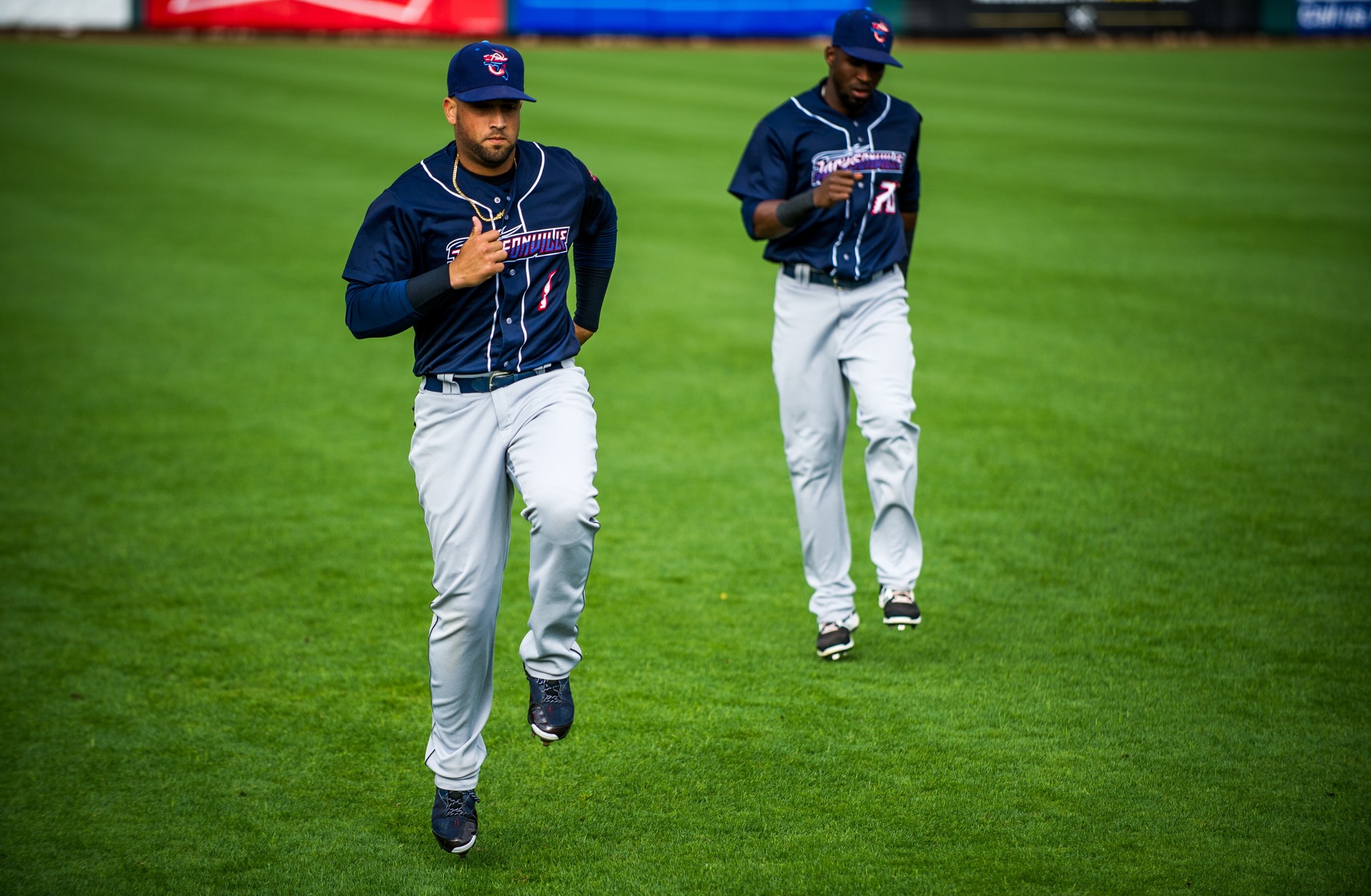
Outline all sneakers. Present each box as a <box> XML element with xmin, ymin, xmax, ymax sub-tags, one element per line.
<box><xmin>817</xmin><ymin>610</ymin><xmax>859</xmax><ymax>661</ymax></box>
<box><xmin>523</xmin><ymin>661</ymin><xmax>574</xmax><ymax>746</ymax></box>
<box><xmin>878</xmin><ymin>585</ymin><xmax>922</xmax><ymax>630</ymax></box>
<box><xmin>429</xmin><ymin>787</ymin><xmax>481</xmax><ymax>859</ymax></box>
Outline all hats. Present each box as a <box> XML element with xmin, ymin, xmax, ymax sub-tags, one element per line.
<box><xmin>447</xmin><ymin>40</ymin><xmax>537</xmax><ymax>102</ymax></box>
<box><xmin>833</xmin><ymin>6</ymin><xmax>903</xmax><ymax>69</ymax></box>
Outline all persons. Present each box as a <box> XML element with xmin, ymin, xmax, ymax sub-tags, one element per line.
<box><xmin>725</xmin><ymin>10</ymin><xmax>923</xmax><ymax>661</ymax></box>
<box><xmin>341</xmin><ymin>41</ymin><xmax>617</xmax><ymax>851</ymax></box>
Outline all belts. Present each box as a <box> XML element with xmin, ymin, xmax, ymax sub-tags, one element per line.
<box><xmin>783</xmin><ymin>262</ymin><xmax>893</xmax><ymax>290</ymax></box>
<box><xmin>424</xmin><ymin>362</ymin><xmax>563</xmax><ymax>393</ymax></box>
